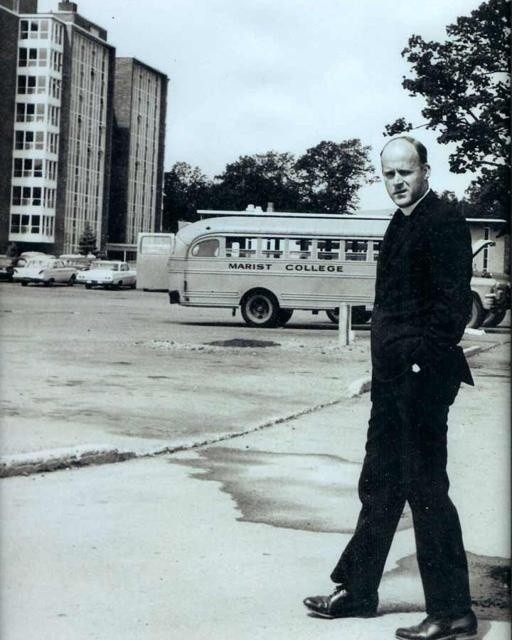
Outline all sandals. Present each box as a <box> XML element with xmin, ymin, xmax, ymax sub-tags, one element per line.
<box><xmin>135</xmin><ymin>210</ymin><xmax>511</xmax><ymax>329</ymax></box>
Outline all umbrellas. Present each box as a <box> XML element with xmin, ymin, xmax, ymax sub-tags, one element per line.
<box><xmin>305</xmin><ymin>586</ymin><xmax>378</xmax><ymax>617</ymax></box>
<box><xmin>396</xmin><ymin>610</ymin><xmax>478</xmax><ymax>640</ymax></box>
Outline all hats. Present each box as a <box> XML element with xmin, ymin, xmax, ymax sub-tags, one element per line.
<box><xmin>0</xmin><ymin>250</ymin><xmax>136</xmax><ymax>290</ymax></box>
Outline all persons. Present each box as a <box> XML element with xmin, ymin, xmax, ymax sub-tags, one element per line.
<box><xmin>302</xmin><ymin>136</ymin><xmax>477</xmax><ymax>640</ymax></box>
